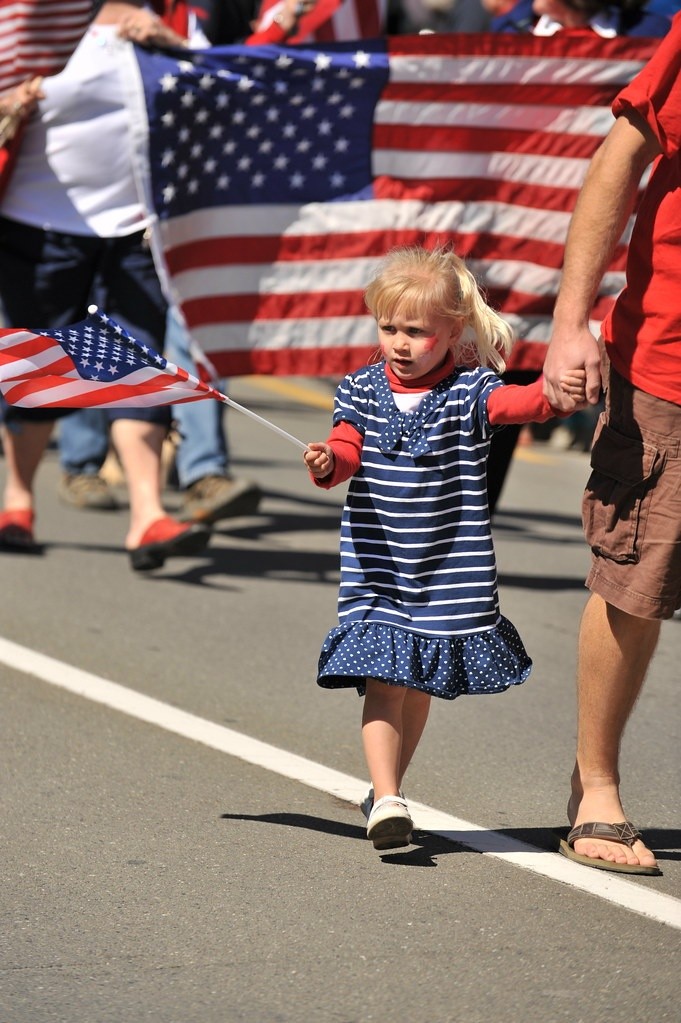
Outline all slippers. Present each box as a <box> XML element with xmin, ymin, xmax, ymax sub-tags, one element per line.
<box><xmin>547</xmin><ymin>821</ymin><xmax>663</xmax><ymax>875</ymax></box>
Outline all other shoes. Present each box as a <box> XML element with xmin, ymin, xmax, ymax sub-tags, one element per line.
<box><xmin>366</xmin><ymin>796</ymin><xmax>414</xmax><ymax>850</ymax></box>
<box><xmin>360</xmin><ymin>788</ymin><xmax>405</xmax><ymax>818</ymax></box>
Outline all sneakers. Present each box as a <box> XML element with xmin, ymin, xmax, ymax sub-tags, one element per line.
<box><xmin>57</xmin><ymin>472</ymin><xmax>117</xmax><ymax>510</ymax></box>
<box><xmin>178</xmin><ymin>475</ymin><xmax>261</xmax><ymax>524</ymax></box>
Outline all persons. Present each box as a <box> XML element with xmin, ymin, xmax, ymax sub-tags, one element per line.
<box><xmin>299</xmin><ymin>251</ymin><xmax>586</xmax><ymax>850</ymax></box>
<box><xmin>0</xmin><ymin>1</ymin><xmax>681</xmax><ymax>572</ymax></box>
<box><xmin>542</xmin><ymin>7</ymin><xmax>681</xmax><ymax>875</ymax></box>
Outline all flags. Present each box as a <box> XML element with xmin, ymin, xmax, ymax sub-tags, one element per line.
<box><xmin>0</xmin><ymin>311</ymin><xmax>228</xmax><ymax>409</ymax></box>
<box><xmin>0</xmin><ymin>0</ymin><xmax>107</xmax><ymax>92</ymax></box>
<box><xmin>114</xmin><ymin>35</ymin><xmax>664</xmax><ymax>378</ymax></box>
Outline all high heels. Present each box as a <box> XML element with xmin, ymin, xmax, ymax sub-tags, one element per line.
<box><xmin>0</xmin><ymin>509</ymin><xmax>35</xmax><ymax>546</ymax></box>
<box><xmin>129</xmin><ymin>517</ymin><xmax>210</xmax><ymax>570</ymax></box>
